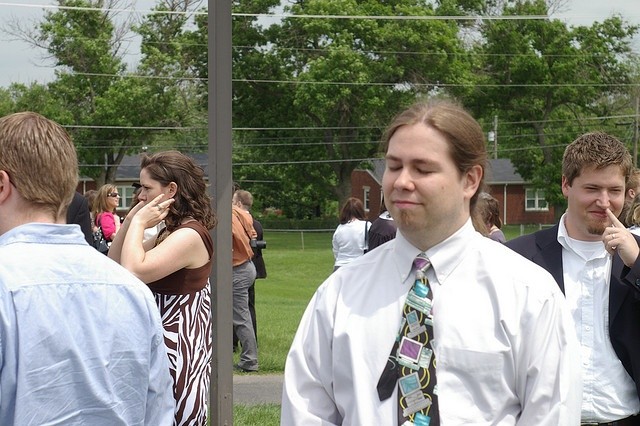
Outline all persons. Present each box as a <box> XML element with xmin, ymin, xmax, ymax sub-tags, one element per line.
<box><xmin>617</xmin><ymin>167</ymin><xmax>640</xmax><ymax>228</ymax></box>
<box><xmin>231</xmin><ymin>189</ymin><xmax>267</xmax><ymax>352</ymax></box>
<box><xmin>279</xmin><ymin>99</ymin><xmax>584</xmax><ymax>426</ymax></box>
<box><xmin>332</xmin><ymin>197</ymin><xmax>373</xmax><ymax>273</ymax></box>
<box><xmin>368</xmin><ymin>195</ymin><xmax>398</xmax><ymax>251</ymax></box>
<box><xmin>0</xmin><ymin>111</ymin><xmax>177</xmax><ymax>426</ymax></box>
<box><xmin>478</xmin><ymin>193</ymin><xmax>507</xmax><ymax>244</ymax></box>
<box><xmin>106</xmin><ymin>149</ymin><xmax>218</xmax><ymax>426</ymax></box>
<box><xmin>84</xmin><ymin>191</ymin><xmax>99</xmax><ymax>233</ymax></box>
<box><xmin>231</xmin><ymin>183</ymin><xmax>259</xmax><ymax>372</ymax></box>
<box><xmin>503</xmin><ymin>131</ymin><xmax>640</xmax><ymax>426</ymax></box>
<box><xmin>91</xmin><ymin>184</ymin><xmax>122</xmax><ymax>247</ymax></box>
<box><xmin>67</xmin><ymin>191</ymin><xmax>92</xmax><ymax>234</ymax></box>
<box><xmin>625</xmin><ymin>193</ymin><xmax>640</xmax><ymax>236</ymax></box>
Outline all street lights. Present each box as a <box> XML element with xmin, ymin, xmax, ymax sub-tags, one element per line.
<box><xmin>488</xmin><ymin>129</ymin><xmax>497</xmax><ymax>159</ymax></box>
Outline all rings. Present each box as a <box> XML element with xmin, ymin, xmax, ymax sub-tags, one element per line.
<box><xmin>612</xmin><ymin>233</ymin><xmax>615</xmax><ymax>239</ymax></box>
<box><xmin>157</xmin><ymin>204</ymin><xmax>162</xmax><ymax>211</ymax></box>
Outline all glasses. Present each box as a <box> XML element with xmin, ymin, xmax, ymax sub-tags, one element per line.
<box><xmin>107</xmin><ymin>192</ymin><xmax>120</xmax><ymax>196</ymax></box>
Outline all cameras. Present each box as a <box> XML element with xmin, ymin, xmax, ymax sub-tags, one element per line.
<box><xmin>250</xmin><ymin>239</ymin><xmax>266</xmax><ymax>249</ymax></box>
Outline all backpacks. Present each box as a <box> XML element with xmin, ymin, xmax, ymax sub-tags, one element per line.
<box><xmin>92</xmin><ymin>211</ymin><xmax>109</xmax><ymax>251</ymax></box>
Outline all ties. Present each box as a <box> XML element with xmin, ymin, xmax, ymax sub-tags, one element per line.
<box><xmin>378</xmin><ymin>253</ymin><xmax>440</xmax><ymax>426</ymax></box>
